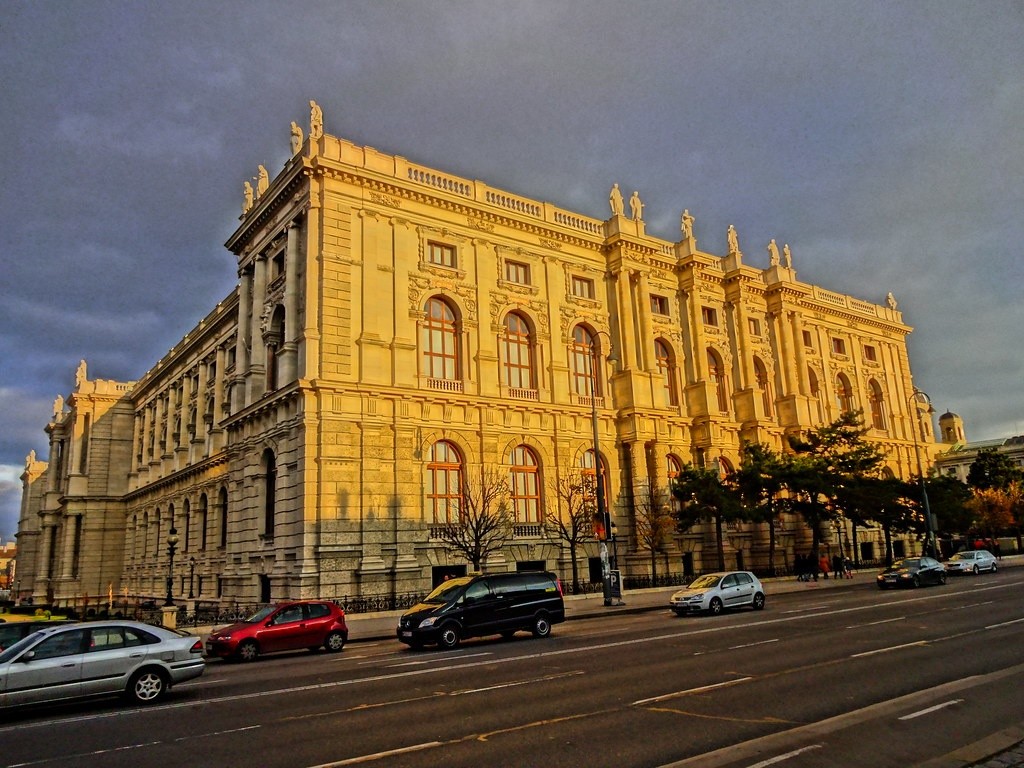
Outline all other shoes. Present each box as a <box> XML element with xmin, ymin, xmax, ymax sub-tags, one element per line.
<box><xmin>814</xmin><ymin>579</ymin><xmax>817</xmax><ymax>582</ymax></box>
<box><xmin>797</xmin><ymin>579</ymin><xmax>800</xmax><ymax>582</ymax></box>
<box><xmin>801</xmin><ymin>578</ymin><xmax>804</xmax><ymax>581</ymax></box>
<box><xmin>804</xmin><ymin>579</ymin><xmax>809</xmax><ymax>582</ymax></box>
<box><xmin>824</xmin><ymin>576</ymin><xmax>829</xmax><ymax>579</ymax></box>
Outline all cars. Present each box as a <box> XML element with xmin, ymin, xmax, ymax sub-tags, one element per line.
<box><xmin>0</xmin><ymin>618</ymin><xmax>208</xmax><ymax>710</ymax></box>
<box><xmin>670</xmin><ymin>570</ymin><xmax>765</xmax><ymax>615</ymax></box>
<box><xmin>877</xmin><ymin>556</ymin><xmax>948</xmax><ymax>588</ymax></box>
<box><xmin>206</xmin><ymin>599</ymin><xmax>348</xmax><ymax>662</ymax></box>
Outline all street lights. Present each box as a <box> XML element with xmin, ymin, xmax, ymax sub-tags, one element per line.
<box><xmin>164</xmin><ymin>525</ymin><xmax>180</xmax><ymax>606</ymax></box>
<box><xmin>836</xmin><ymin>520</ymin><xmax>845</xmax><ymax>560</ymax></box>
<box><xmin>611</xmin><ymin>523</ymin><xmax>625</xmax><ymax>607</ymax></box>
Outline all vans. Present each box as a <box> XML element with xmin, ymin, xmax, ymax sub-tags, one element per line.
<box><xmin>395</xmin><ymin>570</ymin><xmax>568</xmax><ymax>648</ymax></box>
<box><xmin>948</xmin><ymin>551</ymin><xmax>997</xmax><ymax>575</ymax></box>
<box><xmin>0</xmin><ymin>621</ymin><xmax>95</xmax><ymax>662</ymax></box>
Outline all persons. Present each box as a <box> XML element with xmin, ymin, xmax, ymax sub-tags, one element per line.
<box><xmin>610</xmin><ymin>183</ymin><xmax>624</xmax><ymax>216</ymax></box>
<box><xmin>289</xmin><ymin>121</ymin><xmax>303</xmax><ymax>156</ymax></box>
<box><xmin>994</xmin><ymin>541</ymin><xmax>1001</xmax><ymax>560</ymax></box>
<box><xmin>727</xmin><ymin>225</ymin><xmax>741</xmax><ymax>254</ymax></box>
<box><xmin>444</xmin><ymin>573</ymin><xmax>456</xmax><ymax>581</ymax></box>
<box><xmin>630</xmin><ymin>191</ymin><xmax>644</xmax><ymax>220</ymax></box>
<box><xmin>75</xmin><ymin>360</ymin><xmax>86</xmax><ymax>386</ymax></box>
<box><xmin>794</xmin><ymin>552</ymin><xmax>853</xmax><ymax>582</ymax></box>
<box><xmin>252</xmin><ymin>165</ymin><xmax>269</xmax><ymax>199</ymax></box>
<box><xmin>681</xmin><ymin>209</ymin><xmax>695</xmax><ymax>238</ymax></box>
<box><xmin>309</xmin><ymin>101</ymin><xmax>323</xmax><ymax>136</ymax></box>
<box><xmin>25</xmin><ymin>450</ymin><xmax>36</xmax><ymax>466</ymax></box>
<box><xmin>242</xmin><ymin>182</ymin><xmax>254</xmax><ymax>213</ymax></box>
<box><xmin>767</xmin><ymin>239</ymin><xmax>780</xmax><ymax>265</ymax></box>
<box><xmin>52</xmin><ymin>395</ymin><xmax>64</xmax><ymax>422</ymax></box>
<box><xmin>886</xmin><ymin>292</ymin><xmax>897</xmax><ymax>309</ymax></box>
<box><xmin>783</xmin><ymin>243</ymin><xmax>791</xmax><ymax>268</ymax></box>
